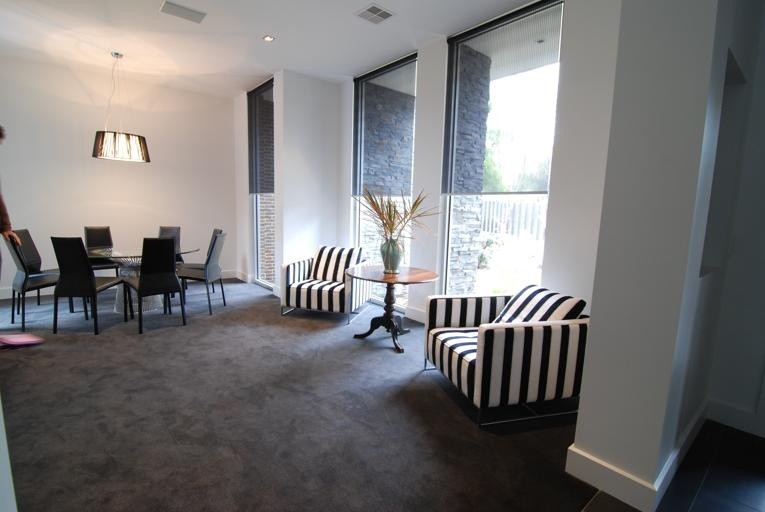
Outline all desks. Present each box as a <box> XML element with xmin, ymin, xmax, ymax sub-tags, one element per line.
<box><xmin>345</xmin><ymin>263</ymin><xmax>440</xmax><ymax>354</ymax></box>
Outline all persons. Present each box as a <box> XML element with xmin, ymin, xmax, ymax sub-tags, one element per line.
<box><xmin>0</xmin><ymin>126</ymin><xmax>23</xmax><ymax>246</ymax></box>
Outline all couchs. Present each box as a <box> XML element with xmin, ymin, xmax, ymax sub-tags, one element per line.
<box><xmin>278</xmin><ymin>244</ymin><xmax>375</xmax><ymax>327</ymax></box>
<box><xmin>418</xmin><ymin>284</ymin><xmax>591</xmax><ymax>434</ymax></box>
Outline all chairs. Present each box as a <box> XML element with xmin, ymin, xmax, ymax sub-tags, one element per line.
<box><xmin>1</xmin><ymin>220</ymin><xmax>229</xmax><ymax>334</ymax></box>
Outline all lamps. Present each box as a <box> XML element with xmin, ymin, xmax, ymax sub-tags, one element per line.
<box><xmin>90</xmin><ymin>52</ymin><xmax>152</xmax><ymax>164</ymax></box>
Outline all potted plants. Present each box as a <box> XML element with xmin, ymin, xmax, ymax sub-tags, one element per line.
<box><xmin>346</xmin><ymin>183</ymin><xmax>444</xmax><ymax>275</ymax></box>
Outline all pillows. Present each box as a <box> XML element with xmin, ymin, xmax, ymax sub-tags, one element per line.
<box><xmin>307</xmin><ymin>244</ymin><xmax>363</xmax><ymax>282</ymax></box>
<box><xmin>489</xmin><ymin>285</ymin><xmax>588</xmax><ymax>322</ymax></box>
